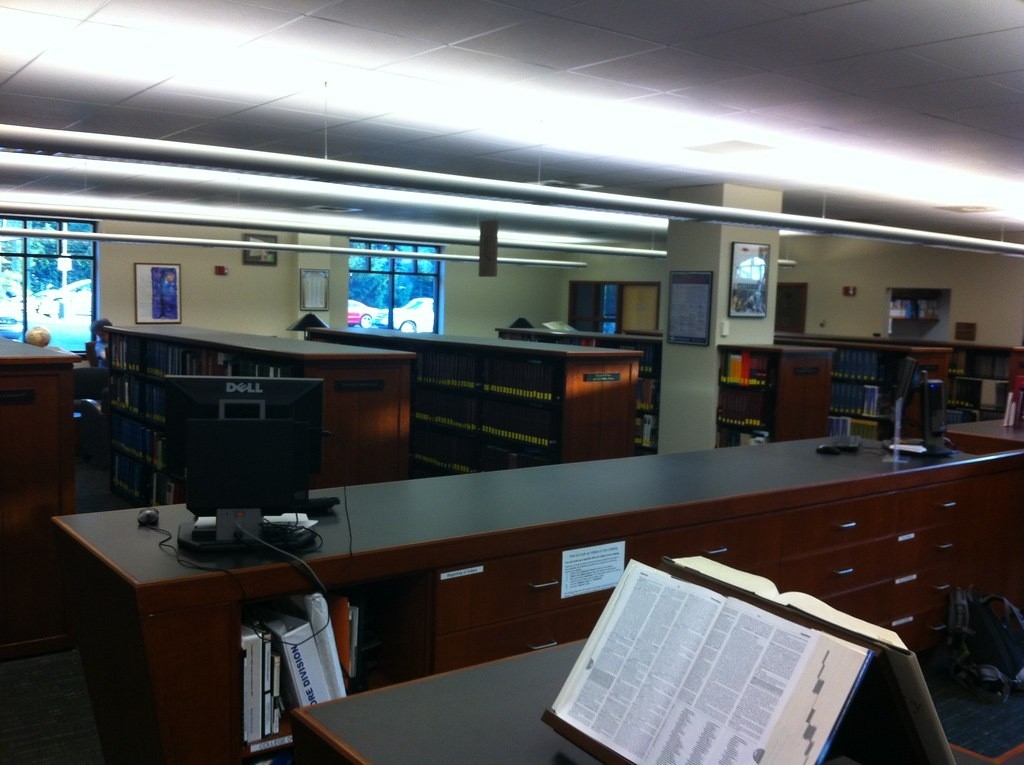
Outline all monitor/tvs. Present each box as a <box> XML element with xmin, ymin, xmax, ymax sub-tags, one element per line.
<box><xmin>894</xmin><ymin>356</ymin><xmax>918</xmax><ymax>406</ymax></box>
<box><xmin>164</xmin><ymin>374</ymin><xmax>323</xmax><ymax>552</ymax></box>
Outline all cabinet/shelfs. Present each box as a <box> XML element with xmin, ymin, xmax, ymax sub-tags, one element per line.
<box><xmin>0</xmin><ymin>326</ymin><xmax>1024</xmax><ymax>765</ymax></box>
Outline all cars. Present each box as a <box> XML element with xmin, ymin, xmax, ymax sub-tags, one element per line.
<box><xmin>0</xmin><ymin>290</ymin><xmax>23</xmax><ymax>340</ymax></box>
<box><xmin>21</xmin><ymin>278</ymin><xmax>91</xmax><ymax>317</ymax></box>
<box><xmin>382</xmin><ymin>297</ymin><xmax>434</xmax><ymax>333</ymax></box>
<box><xmin>348</xmin><ymin>299</ymin><xmax>389</xmax><ymax>328</ymax></box>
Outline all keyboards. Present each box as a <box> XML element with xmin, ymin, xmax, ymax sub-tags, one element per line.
<box><xmin>275</xmin><ymin>497</ymin><xmax>341</xmax><ymax>516</ymax></box>
<box><xmin>824</xmin><ymin>435</ymin><xmax>860</xmax><ymax>451</ymax></box>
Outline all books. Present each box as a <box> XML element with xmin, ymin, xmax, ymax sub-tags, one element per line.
<box><xmin>673</xmin><ymin>555</ymin><xmax>909</xmax><ymax>650</ymax></box>
<box><xmin>110</xmin><ymin>332</ymin><xmax>283</xmax><ymax>510</ymax></box>
<box><xmin>715</xmin><ymin>350</ymin><xmax>769</xmax><ymax>448</ymax></box>
<box><xmin>551</xmin><ymin>559</ymin><xmax>875</xmax><ymax>765</ymax></box>
<box><xmin>945</xmin><ymin>349</ymin><xmax>1009</xmax><ymax>423</ymax></box>
<box><xmin>827</xmin><ymin>349</ymin><xmax>890</xmax><ymax>441</ymax></box>
<box><xmin>262</xmin><ymin>633</ymin><xmax>280</xmax><ymax>737</ymax></box>
<box><xmin>620</xmin><ymin>343</ymin><xmax>661</xmax><ymax>447</ymax></box>
<box><xmin>413</xmin><ymin>351</ymin><xmax>552</xmax><ymax>472</ymax></box>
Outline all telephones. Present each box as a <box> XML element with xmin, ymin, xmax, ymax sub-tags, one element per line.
<box><xmin>921</xmin><ymin>371</ymin><xmax>946</xmax><ymax>448</ymax></box>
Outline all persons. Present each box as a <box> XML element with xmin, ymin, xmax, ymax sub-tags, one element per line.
<box><xmin>90</xmin><ymin>319</ymin><xmax>112</xmax><ymax>367</ymax></box>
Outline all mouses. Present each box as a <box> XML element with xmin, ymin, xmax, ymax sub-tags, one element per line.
<box><xmin>138</xmin><ymin>508</ymin><xmax>159</xmax><ymax>523</ymax></box>
<box><xmin>817</xmin><ymin>444</ymin><xmax>839</xmax><ymax>453</ymax></box>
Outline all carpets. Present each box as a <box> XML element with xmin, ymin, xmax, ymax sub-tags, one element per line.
<box><xmin>925</xmin><ymin>669</ymin><xmax>1024</xmax><ymax>762</ymax></box>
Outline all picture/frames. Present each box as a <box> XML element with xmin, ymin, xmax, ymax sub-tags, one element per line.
<box><xmin>133</xmin><ymin>261</ymin><xmax>182</xmax><ymax>325</ymax></box>
<box><xmin>244</xmin><ymin>234</ymin><xmax>279</xmax><ymax>267</ymax></box>
<box><xmin>666</xmin><ymin>270</ymin><xmax>713</xmax><ymax>346</ymax></box>
<box><xmin>728</xmin><ymin>240</ymin><xmax>770</xmax><ymax>319</ymax></box>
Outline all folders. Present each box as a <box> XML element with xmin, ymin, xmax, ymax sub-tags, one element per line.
<box><xmin>245</xmin><ymin>609</ymin><xmax>330</xmax><ymax>708</ymax></box>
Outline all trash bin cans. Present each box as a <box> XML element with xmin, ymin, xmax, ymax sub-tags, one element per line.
<box><xmin>74</xmin><ymin>399</ymin><xmax>103</xmax><ymax>462</ymax></box>
